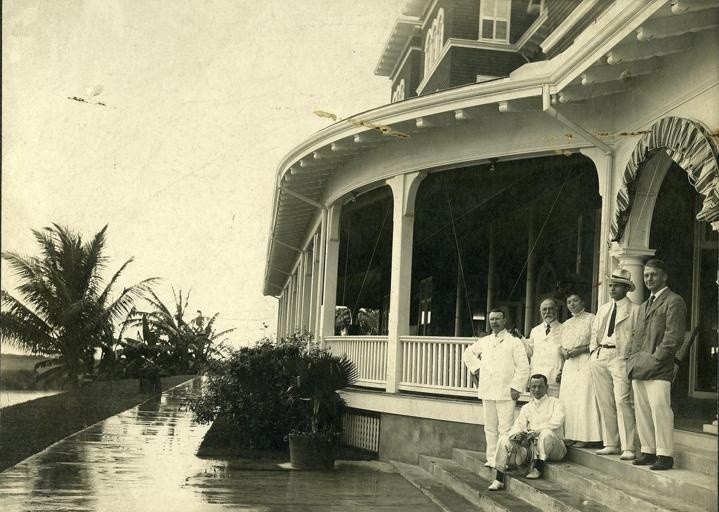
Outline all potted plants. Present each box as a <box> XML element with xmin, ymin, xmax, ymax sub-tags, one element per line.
<box><xmin>286</xmin><ymin>351</ymin><xmax>359</xmax><ymax>472</ymax></box>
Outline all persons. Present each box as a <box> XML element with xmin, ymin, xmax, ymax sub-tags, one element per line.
<box><xmin>513</xmin><ymin>294</ymin><xmax>566</xmax><ymax>398</ymax></box>
<box><xmin>589</xmin><ymin>268</ymin><xmax>641</xmax><ymax>459</ymax></box>
<box><xmin>623</xmin><ymin>258</ymin><xmax>688</xmax><ymax>470</ymax></box>
<box><xmin>488</xmin><ymin>374</ymin><xmax>568</xmax><ymax>491</ymax></box>
<box><xmin>559</xmin><ymin>287</ymin><xmax>603</xmax><ymax>448</ymax></box>
<box><xmin>348</xmin><ymin>308</ymin><xmax>376</xmax><ymax>335</ymax></box>
<box><xmin>463</xmin><ymin>307</ymin><xmax>530</xmax><ymax>470</ymax></box>
<box><xmin>509</xmin><ymin>321</ymin><xmax>518</xmax><ymax>337</ymax></box>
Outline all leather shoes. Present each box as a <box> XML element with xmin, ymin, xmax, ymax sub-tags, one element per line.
<box><xmin>526</xmin><ymin>468</ymin><xmax>543</xmax><ymax>479</ymax></box>
<box><xmin>650</xmin><ymin>456</ymin><xmax>674</xmax><ymax>470</ymax></box>
<box><xmin>594</xmin><ymin>446</ymin><xmax>621</xmax><ymax>455</ymax></box>
<box><xmin>620</xmin><ymin>450</ymin><xmax>635</xmax><ymax>460</ymax></box>
<box><xmin>633</xmin><ymin>453</ymin><xmax>657</xmax><ymax>466</ymax></box>
<box><xmin>489</xmin><ymin>479</ymin><xmax>505</xmax><ymax>491</ymax></box>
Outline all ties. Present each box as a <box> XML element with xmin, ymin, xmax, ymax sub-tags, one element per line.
<box><xmin>607</xmin><ymin>302</ymin><xmax>616</xmax><ymax>336</ymax></box>
<box><xmin>545</xmin><ymin>324</ymin><xmax>551</xmax><ymax>335</ymax></box>
<box><xmin>644</xmin><ymin>295</ymin><xmax>655</xmax><ymax>316</ymax></box>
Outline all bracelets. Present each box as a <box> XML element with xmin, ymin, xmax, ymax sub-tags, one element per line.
<box><xmin>519</xmin><ymin>335</ymin><xmax>523</xmax><ymax>340</ymax></box>
<box><xmin>585</xmin><ymin>345</ymin><xmax>588</xmax><ymax>353</ymax></box>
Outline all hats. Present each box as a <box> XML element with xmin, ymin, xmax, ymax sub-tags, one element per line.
<box><xmin>605</xmin><ymin>268</ymin><xmax>635</xmax><ymax>292</ymax></box>
<box><xmin>515</xmin><ymin>438</ymin><xmax>535</xmax><ymax>474</ymax></box>
